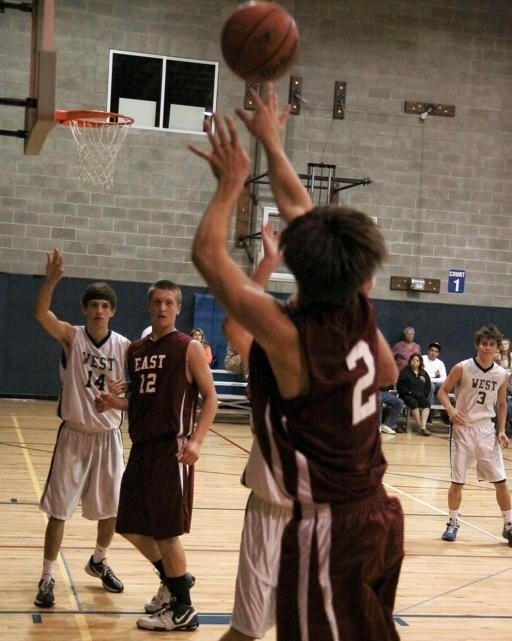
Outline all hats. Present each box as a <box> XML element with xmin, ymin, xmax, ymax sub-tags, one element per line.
<box><xmin>428</xmin><ymin>341</ymin><xmax>442</xmax><ymax>353</ymax></box>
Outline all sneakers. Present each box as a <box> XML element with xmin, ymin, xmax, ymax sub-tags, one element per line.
<box><xmin>441</xmin><ymin>520</ymin><xmax>461</xmax><ymax>542</ymax></box>
<box><xmin>136</xmin><ymin>606</ymin><xmax>201</xmax><ymax>633</ymax></box>
<box><xmin>83</xmin><ymin>556</ymin><xmax>126</xmax><ymax>594</ymax></box>
<box><xmin>143</xmin><ymin>573</ymin><xmax>196</xmax><ymax>614</ymax></box>
<box><xmin>419</xmin><ymin>428</ymin><xmax>432</xmax><ymax>436</ymax></box>
<box><xmin>380</xmin><ymin>424</ymin><xmax>397</xmax><ymax>435</ymax></box>
<box><xmin>33</xmin><ymin>575</ymin><xmax>58</xmax><ymax>609</ymax></box>
<box><xmin>501</xmin><ymin>522</ymin><xmax>512</xmax><ymax>548</ymax></box>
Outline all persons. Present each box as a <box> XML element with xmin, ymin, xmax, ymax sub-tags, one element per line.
<box><xmin>379</xmin><ymin>353</ymin><xmax>407</xmax><ymax>435</ymax></box>
<box><xmin>392</xmin><ymin>326</ymin><xmax>420</xmax><ymax>361</ymax></box>
<box><xmin>396</xmin><ymin>354</ymin><xmax>432</xmax><ymax>437</ymax></box>
<box><xmin>437</xmin><ymin>324</ymin><xmax>512</xmax><ymax>546</ymax></box>
<box><xmin>191</xmin><ymin>328</ymin><xmax>212</xmax><ymax>366</ymax></box>
<box><xmin>494</xmin><ymin>349</ymin><xmax>500</xmax><ymax>366</ymax></box>
<box><xmin>501</xmin><ymin>338</ymin><xmax>512</xmax><ymax>373</ymax></box>
<box><xmin>95</xmin><ymin>279</ymin><xmax>217</xmax><ymax>632</ymax></box>
<box><xmin>186</xmin><ymin>81</ymin><xmax>405</xmax><ymax>641</ymax></box>
<box><xmin>34</xmin><ymin>249</ymin><xmax>131</xmax><ymax>608</ymax></box>
<box><xmin>221</xmin><ymin>223</ymin><xmax>295</xmax><ymax>641</ymax></box>
<box><xmin>422</xmin><ymin>342</ymin><xmax>446</xmax><ymax>423</ymax></box>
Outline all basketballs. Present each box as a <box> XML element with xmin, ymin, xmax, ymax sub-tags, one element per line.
<box><xmin>221</xmin><ymin>1</ymin><xmax>301</xmax><ymax>82</ymax></box>
